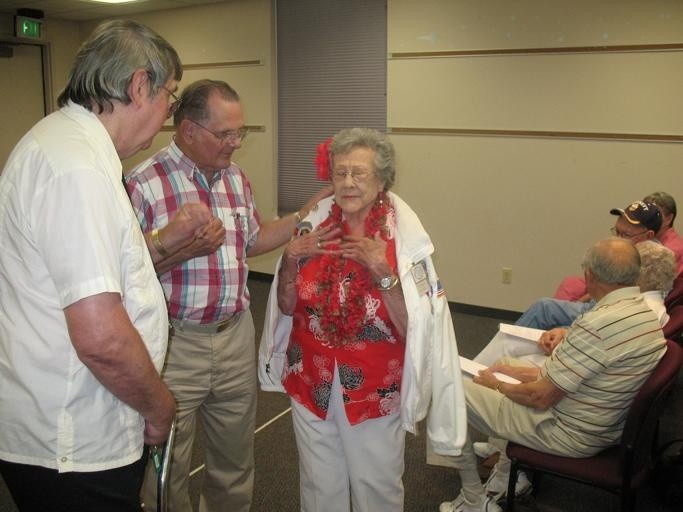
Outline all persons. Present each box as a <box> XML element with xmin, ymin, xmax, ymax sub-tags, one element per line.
<box><xmin>425</xmin><ymin>237</ymin><xmax>667</xmax><ymax>512</ymax></box>
<box><xmin>121</xmin><ymin>78</ymin><xmax>334</xmax><ymax>512</ymax></box>
<box><xmin>260</xmin><ymin>127</ymin><xmax>467</xmax><ymax>512</ymax></box>
<box><xmin>474</xmin><ymin>194</ymin><xmax>683</xmax><ymax>466</ymax></box>
<box><xmin>0</xmin><ymin>18</ymin><xmax>212</xmax><ymax>511</ymax></box>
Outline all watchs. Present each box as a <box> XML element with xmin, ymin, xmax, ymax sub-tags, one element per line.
<box><xmin>373</xmin><ymin>273</ymin><xmax>397</xmax><ymax>288</ymax></box>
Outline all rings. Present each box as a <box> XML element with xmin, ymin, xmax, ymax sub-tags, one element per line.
<box><xmin>318</xmin><ymin>236</ymin><xmax>321</xmax><ymax>241</ymax></box>
<box><xmin>317</xmin><ymin>241</ymin><xmax>321</xmax><ymax>248</ymax></box>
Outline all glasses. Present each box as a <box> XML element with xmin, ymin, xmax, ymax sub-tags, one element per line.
<box><xmin>329</xmin><ymin>169</ymin><xmax>376</xmax><ymax>181</ymax></box>
<box><xmin>185</xmin><ymin>117</ymin><xmax>249</xmax><ymax>146</ymax></box>
<box><xmin>145</xmin><ymin>70</ymin><xmax>184</xmax><ymax>119</ymax></box>
<box><xmin>610</xmin><ymin>226</ymin><xmax>647</xmax><ymax>241</ymax></box>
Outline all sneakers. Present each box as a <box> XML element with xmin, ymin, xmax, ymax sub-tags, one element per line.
<box><xmin>438</xmin><ymin>441</ymin><xmax>535</xmax><ymax>512</ymax></box>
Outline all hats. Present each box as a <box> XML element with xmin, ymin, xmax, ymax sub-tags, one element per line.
<box><xmin>611</xmin><ymin>201</ymin><xmax>662</xmax><ymax>230</ymax></box>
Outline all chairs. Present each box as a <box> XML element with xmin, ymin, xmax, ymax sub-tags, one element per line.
<box><xmin>505</xmin><ymin>269</ymin><xmax>683</xmax><ymax>512</ymax></box>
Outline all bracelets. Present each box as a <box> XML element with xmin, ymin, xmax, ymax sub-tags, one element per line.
<box><xmin>150</xmin><ymin>228</ymin><xmax>171</xmax><ymax>259</ymax></box>
<box><xmin>293</xmin><ymin>211</ymin><xmax>302</xmax><ymax>223</ymax></box>
<box><xmin>377</xmin><ymin>279</ymin><xmax>399</xmax><ymax>291</ymax></box>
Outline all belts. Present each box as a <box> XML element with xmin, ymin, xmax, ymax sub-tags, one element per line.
<box><xmin>169</xmin><ymin>312</ymin><xmax>242</xmax><ymax>334</ymax></box>
